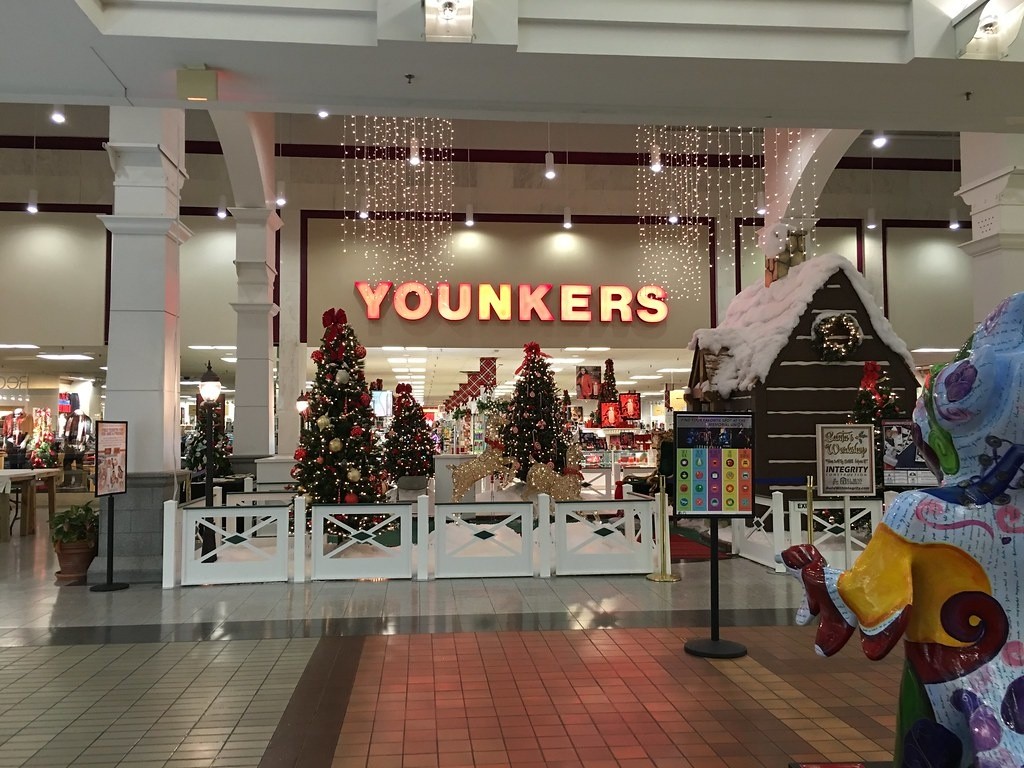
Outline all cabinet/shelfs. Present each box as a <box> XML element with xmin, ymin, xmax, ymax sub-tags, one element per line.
<box><xmin>433</xmin><ymin>454</ymin><xmax>477</xmax><ymax>503</ymax></box>
<box><xmin>0</xmin><ymin>420</ymin><xmax>5</xmax><ymax>429</ymax></box>
<box><xmin>459</xmin><ymin>415</ymin><xmax>487</xmax><ymax>457</ymax></box>
<box><xmin>255</xmin><ymin>455</ymin><xmax>302</xmax><ymax>537</ymax></box>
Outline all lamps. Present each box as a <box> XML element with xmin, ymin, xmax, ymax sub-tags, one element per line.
<box><xmin>757</xmin><ymin>129</ymin><xmax>766</xmax><ymax>214</ymax></box>
<box><xmin>949</xmin><ymin>133</ymin><xmax>959</xmax><ymax>229</ymax></box>
<box><xmin>651</xmin><ymin>125</ymin><xmax>662</xmax><ymax>171</ymax></box>
<box><xmin>296</xmin><ymin>390</ymin><xmax>308</xmax><ymax>413</ymax></box>
<box><xmin>176</xmin><ymin>64</ymin><xmax>223</xmax><ymax>102</ymax></box>
<box><xmin>361</xmin><ymin>196</ymin><xmax>369</xmax><ymax>218</ymax></box>
<box><xmin>410</xmin><ymin>117</ymin><xmax>420</xmax><ymax>166</ymax></box>
<box><xmin>53</xmin><ymin>105</ymin><xmax>65</xmax><ymax>123</ymax></box>
<box><xmin>276</xmin><ymin>113</ymin><xmax>286</xmax><ymax>206</ymax></box>
<box><xmin>951</xmin><ymin>0</ymin><xmax>1024</xmax><ymax>61</ymax></box>
<box><xmin>545</xmin><ymin>120</ymin><xmax>555</xmax><ymax>179</ymax></box>
<box><xmin>564</xmin><ymin>150</ymin><xmax>572</xmax><ymax>229</ymax></box>
<box><xmin>873</xmin><ymin>129</ymin><xmax>886</xmax><ymax>148</ymax></box>
<box><xmin>28</xmin><ymin>104</ymin><xmax>38</xmax><ymax>213</ymax></box>
<box><xmin>669</xmin><ymin>198</ymin><xmax>678</xmax><ymax>223</ymax></box>
<box><xmin>866</xmin><ymin>149</ymin><xmax>877</xmax><ymax>229</ymax></box>
<box><xmin>198</xmin><ymin>361</ymin><xmax>221</xmax><ymax>401</ymax></box>
<box><xmin>421</xmin><ymin>0</ymin><xmax>475</xmax><ymax>43</ymax></box>
<box><xmin>466</xmin><ymin>148</ymin><xmax>473</xmax><ymax>227</ymax></box>
<box><xmin>218</xmin><ymin>196</ymin><xmax>226</xmax><ymax>219</ymax></box>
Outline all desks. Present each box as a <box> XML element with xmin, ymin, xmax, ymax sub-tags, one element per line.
<box><xmin>0</xmin><ymin>468</ymin><xmax>61</xmax><ymax>536</ymax></box>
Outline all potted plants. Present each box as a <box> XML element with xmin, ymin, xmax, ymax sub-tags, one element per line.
<box><xmin>46</xmin><ymin>501</ymin><xmax>100</xmax><ymax>575</ymax></box>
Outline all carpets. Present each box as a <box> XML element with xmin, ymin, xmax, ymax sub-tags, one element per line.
<box><xmin>328</xmin><ymin>514</ymin><xmax>733</xmax><ymax>563</ymax></box>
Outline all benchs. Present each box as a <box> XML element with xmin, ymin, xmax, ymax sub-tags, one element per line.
<box><xmin>623</xmin><ymin>466</ymin><xmax>657</xmax><ymax>477</ymax></box>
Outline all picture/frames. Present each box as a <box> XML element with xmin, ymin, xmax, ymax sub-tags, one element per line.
<box><xmin>619</xmin><ymin>393</ymin><xmax>641</xmax><ymax>421</ymax></box>
<box><xmin>600</xmin><ymin>401</ymin><xmax>619</xmax><ymax>427</ymax></box>
<box><xmin>620</xmin><ymin>432</ymin><xmax>635</xmax><ymax>446</ymax></box>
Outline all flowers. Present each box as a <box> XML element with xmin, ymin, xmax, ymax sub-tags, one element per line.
<box><xmin>847</xmin><ymin>361</ymin><xmax>900</xmax><ymax>425</ymax></box>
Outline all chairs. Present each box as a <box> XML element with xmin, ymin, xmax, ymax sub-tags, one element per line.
<box><xmin>397</xmin><ymin>476</ymin><xmax>428</xmax><ymax>503</ymax></box>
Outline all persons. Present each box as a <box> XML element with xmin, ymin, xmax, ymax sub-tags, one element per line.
<box><xmin>59</xmin><ymin>409</ymin><xmax>92</xmax><ymax>487</ymax></box>
<box><xmin>576</xmin><ymin>367</ymin><xmax>592</xmax><ymax>400</ymax></box>
<box><xmin>2</xmin><ymin>408</ymin><xmax>34</xmax><ymax>470</ymax></box>
<box><xmin>775</xmin><ymin>292</ymin><xmax>1024</xmax><ymax>768</ymax></box>
